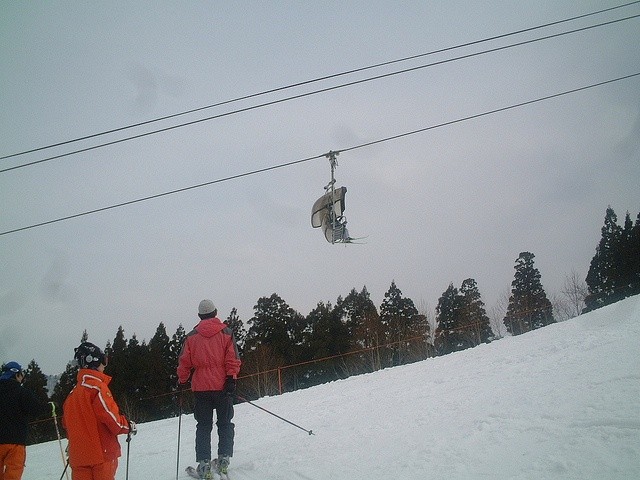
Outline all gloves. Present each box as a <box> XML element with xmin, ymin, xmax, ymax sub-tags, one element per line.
<box><xmin>48</xmin><ymin>402</ymin><xmax>55</xmax><ymax>417</ymax></box>
<box><xmin>128</xmin><ymin>421</ymin><xmax>137</xmax><ymax>435</ymax></box>
<box><xmin>224</xmin><ymin>375</ymin><xmax>236</xmax><ymax>394</ymax></box>
<box><xmin>177</xmin><ymin>382</ymin><xmax>186</xmax><ymax>390</ymax></box>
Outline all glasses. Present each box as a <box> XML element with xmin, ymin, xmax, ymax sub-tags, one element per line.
<box><xmin>101</xmin><ymin>354</ymin><xmax>109</xmax><ymax>365</ymax></box>
<box><xmin>2</xmin><ymin>368</ymin><xmax>20</xmax><ymax>373</ymax></box>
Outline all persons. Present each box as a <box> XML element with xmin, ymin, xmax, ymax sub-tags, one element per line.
<box><xmin>331</xmin><ymin>211</ymin><xmax>353</xmax><ymax>244</ymax></box>
<box><xmin>0</xmin><ymin>361</ymin><xmax>56</xmax><ymax>480</ymax></box>
<box><xmin>176</xmin><ymin>299</ymin><xmax>241</xmax><ymax>478</ymax></box>
<box><xmin>61</xmin><ymin>342</ymin><xmax>137</xmax><ymax>480</ymax></box>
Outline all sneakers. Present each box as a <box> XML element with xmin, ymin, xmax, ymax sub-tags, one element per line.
<box><xmin>217</xmin><ymin>455</ymin><xmax>231</xmax><ymax>472</ymax></box>
<box><xmin>344</xmin><ymin>236</ymin><xmax>350</xmax><ymax>239</ymax></box>
<box><xmin>197</xmin><ymin>461</ymin><xmax>211</xmax><ymax>477</ymax></box>
<box><xmin>344</xmin><ymin>240</ymin><xmax>350</xmax><ymax>242</ymax></box>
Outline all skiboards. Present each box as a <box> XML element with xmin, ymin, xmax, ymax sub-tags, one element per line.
<box><xmin>185</xmin><ymin>459</ymin><xmax>230</xmax><ymax>480</ymax></box>
<box><xmin>334</xmin><ymin>236</ymin><xmax>368</xmax><ymax>244</ymax></box>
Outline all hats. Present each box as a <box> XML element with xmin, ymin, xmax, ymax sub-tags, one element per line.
<box><xmin>0</xmin><ymin>362</ymin><xmax>21</xmax><ymax>380</ymax></box>
<box><xmin>198</xmin><ymin>299</ymin><xmax>217</xmax><ymax>314</ymax></box>
<box><xmin>74</xmin><ymin>342</ymin><xmax>105</xmax><ymax>369</ymax></box>
